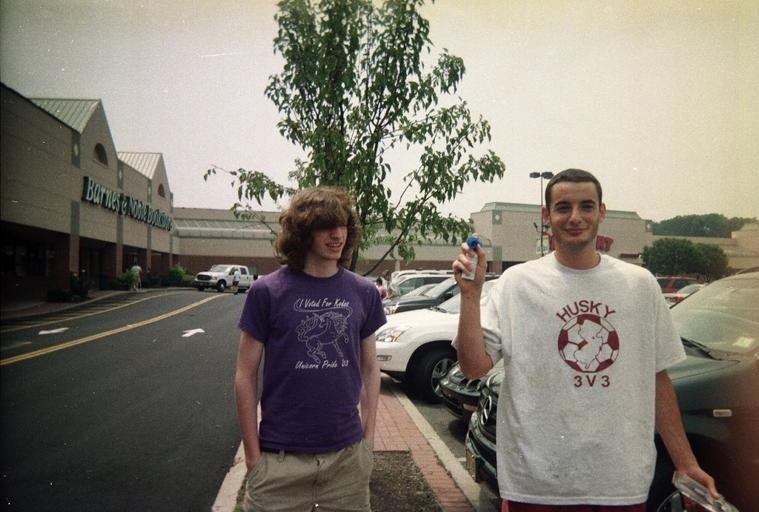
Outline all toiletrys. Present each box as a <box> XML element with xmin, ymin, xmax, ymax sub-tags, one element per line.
<box><xmin>461</xmin><ymin>233</ymin><xmax>480</xmax><ymax>282</ymax></box>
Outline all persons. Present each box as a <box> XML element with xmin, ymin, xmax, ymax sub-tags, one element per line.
<box><xmin>131</xmin><ymin>262</ymin><xmax>143</xmax><ymax>291</ymax></box>
<box><xmin>452</xmin><ymin>168</ymin><xmax>720</xmax><ymax>512</ymax></box>
<box><xmin>232</xmin><ymin>268</ymin><xmax>241</xmax><ymax>295</ymax></box>
<box><xmin>234</xmin><ymin>188</ymin><xmax>388</xmax><ymax>511</ymax></box>
<box><xmin>250</xmin><ymin>272</ymin><xmax>258</xmax><ymax>291</ymax></box>
<box><xmin>375</xmin><ymin>278</ymin><xmax>386</xmax><ymax>301</ymax></box>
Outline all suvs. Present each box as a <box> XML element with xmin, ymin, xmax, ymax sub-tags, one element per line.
<box><xmin>457</xmin><ymin>266</ymin><xmax>759</xmax><ymax>507</ymax></box>
<box><xmin>375</xmin><ymin>279</ymin><xmax>500</xmax><ymax>406</ymax></box>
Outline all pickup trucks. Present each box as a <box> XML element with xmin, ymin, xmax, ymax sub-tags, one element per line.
<box><xmin>195</xmin><ymin>263</ymin><xmax>264</xmax><ymax>292</ymax></box>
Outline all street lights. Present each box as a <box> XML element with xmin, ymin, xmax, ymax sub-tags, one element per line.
<box><xmin>527</xmin><ymin>171</ymin><xmax>555</xmax><ymax>257</ymax></box>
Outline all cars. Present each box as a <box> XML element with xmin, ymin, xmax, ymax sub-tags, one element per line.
<box><xmin>434</xmin><ymin>356</ymin><xmax>484</xmax><ymax>426</ymax></box>
<box><xmin>656</xmin><ymin>276</ymin><xmax>703</xmax><ymax>294</ymax></box>
<box><xmin>662</xmin><ymin>282</ymin><xmax>706</xmax><ymax>309</ymax></box>
<box><xmin>377</xmin><ymin>266</ymin><xmax>500</xmax><ymax>312</ymax></box>
